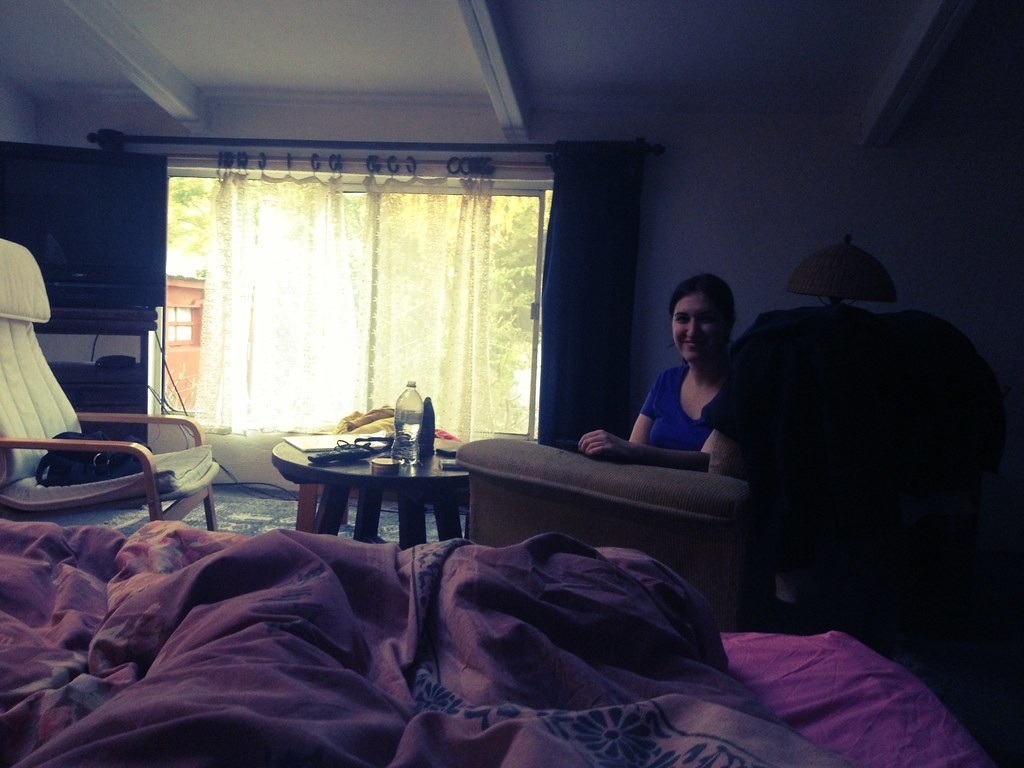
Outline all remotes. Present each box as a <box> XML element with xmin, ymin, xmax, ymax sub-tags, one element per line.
<box><xmin>308</xmin><ymin>447</ymin><xmax>372</xmax><ymax>463</ymax></box>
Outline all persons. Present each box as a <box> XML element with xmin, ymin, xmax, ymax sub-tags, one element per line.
<box><xmin>578</xmin><ymin>274</ymin><xmax>738</xmax><ymax>472</ymax></box>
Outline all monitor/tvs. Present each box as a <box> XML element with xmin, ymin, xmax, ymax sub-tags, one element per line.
<box><xmin>0</xmin><ymin>140</ymin><xmax>168</xmax><ymax>309</ymax></box>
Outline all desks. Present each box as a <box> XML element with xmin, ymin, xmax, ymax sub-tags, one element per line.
<box><xmin>271</xmin><ymin>432</ymin><xmax>469</xmax><ymax>548</ymax></box>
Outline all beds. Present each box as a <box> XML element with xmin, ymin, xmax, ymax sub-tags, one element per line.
<box><xmin>0</xmin><ymin>516</ymin><xmax>1002</xmax><ymax>768</ymax></box>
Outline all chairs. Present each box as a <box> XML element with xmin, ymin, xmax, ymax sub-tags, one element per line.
<box><xmin>459</xmin><ymin>442</ymin><xmax>751</xmax><ymax>632</ymax></box>
<box><xmin>0</xmin><ymin>239</ymin><xmax>218</xmax><ymax>537</ymax></box>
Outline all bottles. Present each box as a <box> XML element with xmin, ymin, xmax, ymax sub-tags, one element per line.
<box><xmin>418</xmin><ymin>397</ymin><xmax>435</xmax><ymax>456</ymax></box>
<box><xmin>391</xmin><ymin>381</ymin><xmax>423</xmax><ymax>465</ymax></box>
